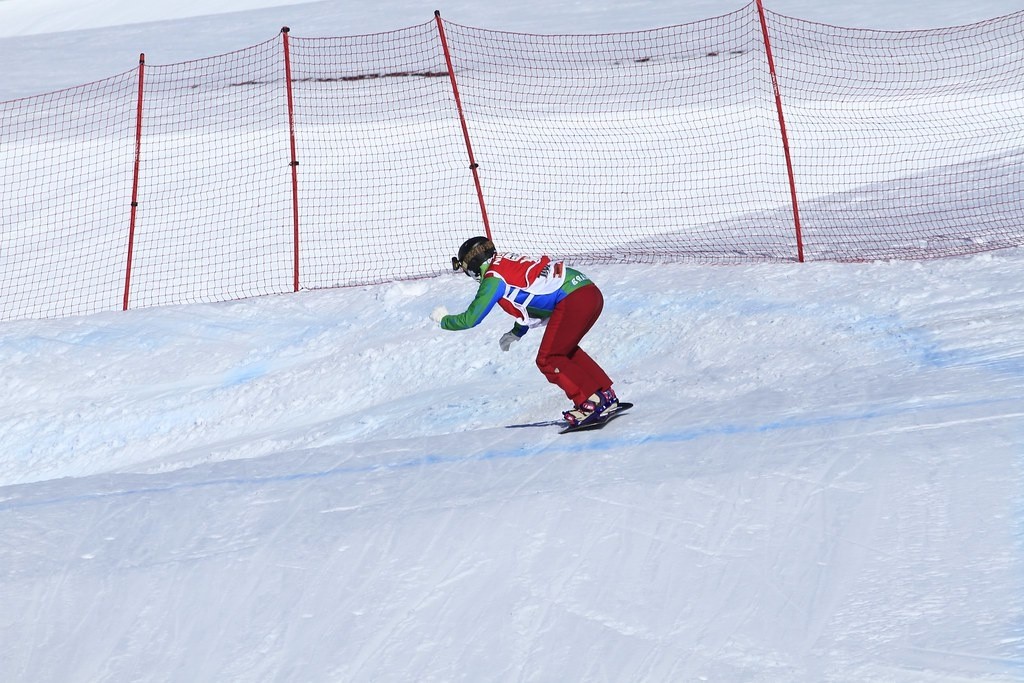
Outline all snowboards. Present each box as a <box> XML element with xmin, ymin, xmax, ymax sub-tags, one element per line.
<box><xmin>558</xmin><ymin>399</ymin><xmax>634</xmax><ymax>434</ymax></box>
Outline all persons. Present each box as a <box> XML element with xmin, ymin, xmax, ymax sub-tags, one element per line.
<box><xmin>427</xmin><ymin>235</ymin><xmax>618</xmax><ymax>428</ymax></box>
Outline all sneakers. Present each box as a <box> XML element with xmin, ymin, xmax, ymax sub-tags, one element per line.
<box><xmin>598</xmin><ymin>389</ymin><xmax>618</xmax><ymax>411</ymax></box>
<box><xmin>562</xmin><ymin>390</ymin><xmax>608</xmax><ymax>420</ymax></box>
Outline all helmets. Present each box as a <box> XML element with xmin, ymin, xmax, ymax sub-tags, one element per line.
<box><xmin>459</xmin><ymin>236</ymin><xmax>496</xmax><ymax>277</ymax></box>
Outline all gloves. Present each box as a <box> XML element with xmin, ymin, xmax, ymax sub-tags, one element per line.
<box><xmin>499</xmin><ymin>331</ymin><xmax>520</xmax><ymax>351</ymax></box>
<box><xmin>429</xmin><ymin>306</ymin><xmax>449</xmax><ymax>325</ymax></box>
